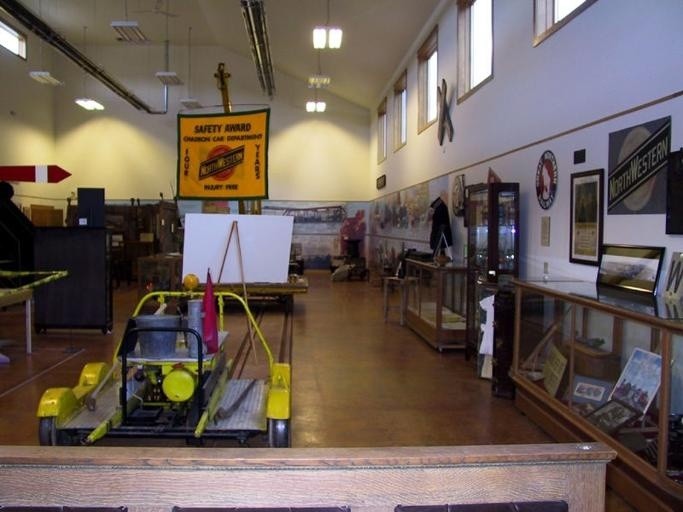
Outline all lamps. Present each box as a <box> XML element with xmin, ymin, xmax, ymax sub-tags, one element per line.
<box><xmin>109</xmin><ymin>0</ymin><xmax>204</xmax><ymax>109</ymax></box>
<box><xmin>304</xmin><ymin>2</ymin><xmax>343</xmax><ymax>112</ymax></box>
<box><xmin>25</xmin><ymin>22</ymin><xmax>105</xmax><ymax>112</ymax></box>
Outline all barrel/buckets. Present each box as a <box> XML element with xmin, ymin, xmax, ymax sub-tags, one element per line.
<box><xmin>133</xmin><ymin>313</ymin><xmax>181</xmax><ymax>359</ymax></box>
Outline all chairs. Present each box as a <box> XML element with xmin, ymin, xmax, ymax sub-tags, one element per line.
<box><xmin>346</xmin><ymin>256</ymin><xmax>370</xmax><ymax>284</ymax></box>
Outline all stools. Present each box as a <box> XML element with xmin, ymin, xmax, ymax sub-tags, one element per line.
<box><xmin>382</xmin><ymin>275</ymin><xmax>420</xmax><ymax>326</ymax></box>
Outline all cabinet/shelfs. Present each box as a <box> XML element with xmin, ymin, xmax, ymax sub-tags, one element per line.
<box><xmin>508</xmin><ymin>273</ymin><xmax>681</xmax><ymax>512</ymax></box>
<box><xmin>462</xmin><ymin>182</ymin><xmax>520</xmax><ymax>368</ymax></box>
<box><xmin>398</xmin><ymin>253</ymin><xmax>467</xmax><ymax>353</ymax></box>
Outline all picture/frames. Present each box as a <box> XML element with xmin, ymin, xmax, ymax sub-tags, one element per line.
<box><xmin>568</xmin><ymin>167</ymin><xmax>603</xmax><ymax>267</ymax></box>
